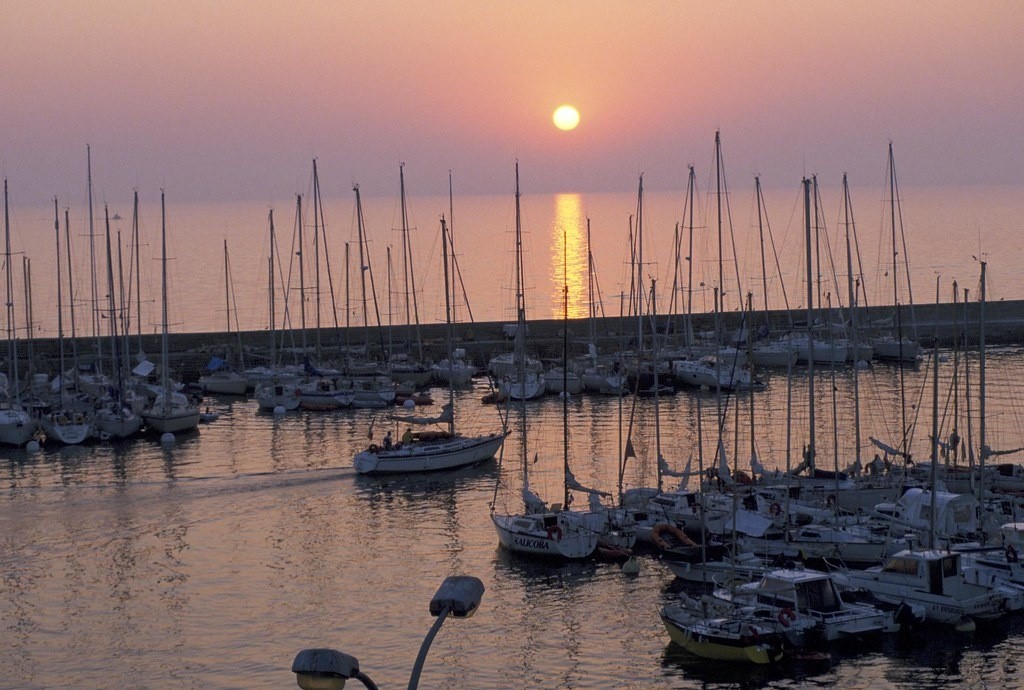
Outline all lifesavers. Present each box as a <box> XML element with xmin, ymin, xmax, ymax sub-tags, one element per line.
<box><xmin>770</xmin><ymin>503</ymin><xmax>781</xmax><ymax>516</ymax></box>
<box><xmin>778</xmin><ymin>608</ymin><xmax>796</xmax><ymax>628</ymax></box>
<box><xmin>1005</xmin><ymin>544</ymin><xmax>1018</xmax><ymax>563</ymax></box>
<box><xmin>369</xmin><ymin>444</ymin><xmax>378</xmax><ymax>452</ymax></box>
<box><xmin>547</xmin><ymin>525</ymin><xmax>562</xmax><ymax>540</ymax></box>
<box><xmin>190</xmin><ymin>395</ymin><xmax>200</xmax><ymax>406</ymax></box>
<box><xmin>827</xmin><ymin>494</ymin><xmax>836</xmax><ymax>506</ymax></box>
<box><xmin>503</xmin><ymin>376</ymin><xmax>510</xmax><ymax>383</ymax></box>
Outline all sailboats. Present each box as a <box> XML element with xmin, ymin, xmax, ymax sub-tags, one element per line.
<box><xmin>0</xmin><ymin>129</ymin><xmax>1024</xmax><ymax>675</ymax></box>
<box><xmin>349</xmin><ymin>212</ymin><xmax>514</xmax><ymax>475</ymax></box>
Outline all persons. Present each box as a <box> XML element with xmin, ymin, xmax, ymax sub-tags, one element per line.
<box><xmin>402</xmin><ymin>428</ymin><xmax>414</xmax><ymax>446</ymax></box>
<box><xmin>800</xmin><ymin>443</ymin><xmax>817</xmax><ymax>475</ymax></box>
<box><xmin>711</xmin><ymin>469</ymin><xmax>756</xmax><ymax>484</ymax></box>
<box><xmin>865</xmin><ymin>455</ymin><xmax>885</xmax><ymax>475</ymax></box>
<box><xmin>382</xmin><ymin>431</ymin><xmax>392</xmax><ymax>451</ymax></box>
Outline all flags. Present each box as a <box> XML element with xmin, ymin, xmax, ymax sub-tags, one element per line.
<box><xmin>624</xmin><ymin>440</ymin><xmax>638</xmax><ymax>460</ymax></box>
<box><xmin>795</xmin><ymin>549</ymin><xmax>809</xmax><ymax>561</ymax></box>
<box><xmin>302</xmin><ymin>356</ymin><xmax>324</xmax><ymax>377</ymax></box>
